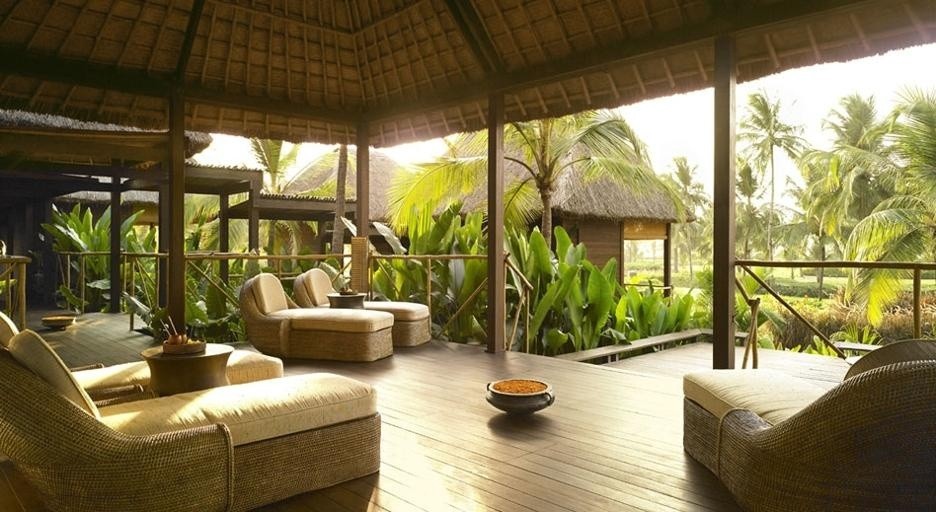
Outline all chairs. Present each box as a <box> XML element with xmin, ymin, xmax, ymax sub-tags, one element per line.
<box><xmin>293</xmin><ymin>272</ymin><xmax>432</xmax><ymax>353</ymax></box>
<box><xmin>0</xmin><ymin>348</ymin><xmax>381</xmax><ymax>511</ymax></box>
<box><xmin>683</xmin><ymin>359</ymin><xmax>936</xmax><ymax>511</ymax></box>
<box><xmin>0</xmin><ymin>312</ymin><xmax>283</xmax><ymax>406</ymax></box>
<box><xmin>239</xmin><ymin>278</ymin><xmax>394</xmax><ymax>367</ymax></box>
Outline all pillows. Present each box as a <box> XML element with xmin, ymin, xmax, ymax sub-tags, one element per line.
<box><xmin>0</xmin><ymin>312</ymin><xmax>19</xmax><ymax>347</ymax></box>
<box><xmin>7</xmin><ymin>329</ymin><xmax>101</xmax><ymax>422</ymax></box>
<box><xmin>843</xmin><ymin>339</ymin><xmax>936</xmax><ymax>379</ymax></box>
<box><xmin>252</xmin><ymin>272</ymin><xmax>288</xmax><ymax>314</ymax></box>
<box><xmin>302</xmin><ymin>268</ymin><xmax>332</xmax><ymax>305</ymax></box>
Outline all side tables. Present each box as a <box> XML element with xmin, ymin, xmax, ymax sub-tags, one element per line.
<box><xmin>141</xmin><ymin>343</ymin><xmax>236</xmax><ymax>397</ymax></box>
<box><xmin>326</xmin><ymin>293</ymin><xmax>367</xmax><ymax>308</ymax></box>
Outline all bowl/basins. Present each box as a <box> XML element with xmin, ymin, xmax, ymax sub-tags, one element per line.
<box><xmin>485</xmin><ymin>378</ymin><xmax>556</xmax><ymax>418</ymax></box>
<box><xmin>40</xmin><ymin>314</ymin><xmax>74</xmax><ymax>327</ymax></box>
<box><xmin>162</xmin><ymin>339</ymin><xmax>208</xmax><ymax>355</ymax></box>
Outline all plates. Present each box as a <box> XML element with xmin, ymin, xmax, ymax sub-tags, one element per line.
<box><xmin>339</xmin><ymin>291</ymin><xmax>358</xmax><ymax>296</ymax></box>
